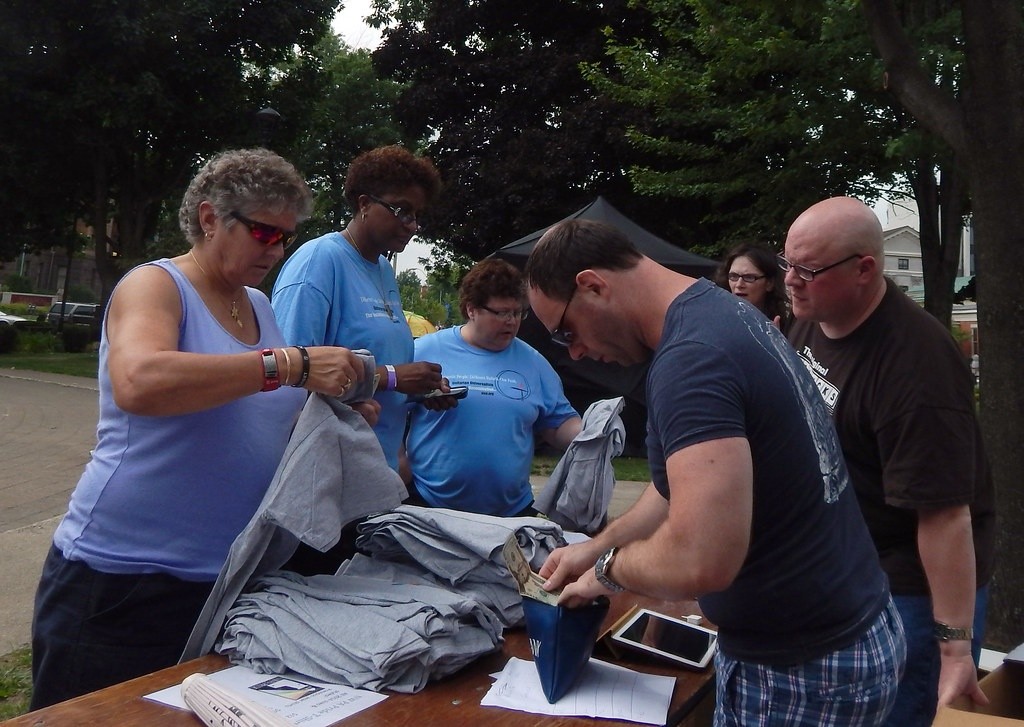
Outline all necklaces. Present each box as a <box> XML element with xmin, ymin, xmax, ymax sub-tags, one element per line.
<box><xmin>189</xmin><ymin>250</ymin><xmax>246</xmax><ymax>327</ymax></box>
<box><xmin>346</xmin><ymin>228</ymin><xmax>393</xmax><ymax>317</ymax></box>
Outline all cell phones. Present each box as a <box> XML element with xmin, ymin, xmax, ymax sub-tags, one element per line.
<box><xmin>427</xmin><ymin>387</ymin><xmax>469</xmax><ymax>399</ymax></box>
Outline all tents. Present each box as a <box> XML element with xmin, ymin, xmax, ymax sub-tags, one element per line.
<box><xmin>486</xmin><ymin>196</ymin><xmax>725</xmax><ymax>458</ymax></box>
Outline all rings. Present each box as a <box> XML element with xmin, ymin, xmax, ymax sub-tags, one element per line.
<box><xmin>337</xmin><ymin>388</ymin><xmax>344</xmax><ymax>397</ymax></box>
<box><xmin>343</xmin><ymin>378</ymin><xmax>352</xmax><ymax>389</ymax></box>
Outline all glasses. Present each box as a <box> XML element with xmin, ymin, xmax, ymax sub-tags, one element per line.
<box><xmin>371</xmin><ymin>195</ymin><xmax>425</xmax><ymax>234</ymax></box>
<box><xmin>728</xmin><ymin>272</ymin><xmax>767</xmax><ymax>282</ymax></box>
<box><xmin>553</xmin><ymin>285</ymin><xmax>578</xmax><ymax>347</ymax></box>
<box><xmin>229</xmin><ymin>212</ymin><xmax>299</xmax><ymax>250</ymax></box>
<box><xmin>482</xmin><ymin>306</ymin><xmax>528</xmax><ymax>321</ymax></box>
<box><xmin>776</xmin><ymin>250</ymin><xmax>862</xmax><ymax>282</ymax></box>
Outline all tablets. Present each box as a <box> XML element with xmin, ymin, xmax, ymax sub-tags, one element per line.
<box><xmin>613</xmin><ymin>609</ymin><xmax>718</xmax><ymax>668</ymax></box>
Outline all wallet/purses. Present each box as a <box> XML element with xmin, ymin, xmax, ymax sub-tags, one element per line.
<box><xmin>521</xmin><ymin>594</ymin><xmax>611</xmax><ymax>703</ymax></box>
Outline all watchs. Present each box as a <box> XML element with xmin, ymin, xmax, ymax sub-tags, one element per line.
<box><xmin>595</xmin><ymin>546</ymin><xmax>627</xmax><ymax>592</ymax></box>
<box><xmin>933</xmin><ymin>620</ymin><xmax>973</xmax><ymax>642</ymax></box>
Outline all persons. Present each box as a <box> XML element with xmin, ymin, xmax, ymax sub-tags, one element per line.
<box><xmin>272</xmin><ymin>145</ymin><xmax>458</xmax><ymax>576</ymax></box>
<box><xmin>29</xmin><ymin>149</ymin><xmax>382</xmax><ymax>712</ymax></box>
<box><xmin>781</xmin><ymin>196</ymin><xmax>997</xmax><ymax>727</ymax></box>
<box><xmin>640</xmin><ymin>614</ymin><xmax>676</xmax><ymax>653</ymax></box>
<box><xmin>399</xmin><ymin>260</ymin><xmax>582</xmax><ymax>517</ymax></box>
<box><xmin>714</xmin><ymin>242</ymin><xmax>792</xmax><ymax>332</ymax></box>
<box><xmin>522</xmin><ymin>220</ymin><xmax>907</xmax><ymax>727</ymax></box>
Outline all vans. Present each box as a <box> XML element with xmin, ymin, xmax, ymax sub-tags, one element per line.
<box><xmin>46</xmin><ymin>302</ymin><xmax>101</xmax><ymax>324</ymax></box>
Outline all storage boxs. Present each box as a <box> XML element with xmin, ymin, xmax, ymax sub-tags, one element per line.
<box><xmin>932</xmin><ymin>664</ymin><xmax>1024</xmax><ymax>727</ymax></box>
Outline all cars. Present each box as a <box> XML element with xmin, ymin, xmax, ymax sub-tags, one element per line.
<box><xmin>0</xmin><ymin>311</ymin><xmax>26</xmax><ymax>328</ymax></box>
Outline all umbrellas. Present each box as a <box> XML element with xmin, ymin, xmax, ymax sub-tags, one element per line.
<box><xmin>402</xmin><ymin>310</ymin><xmax>437</xmax><ymax>337</ymax></box>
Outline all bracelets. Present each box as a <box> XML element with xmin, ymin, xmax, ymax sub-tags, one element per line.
<box><xmin>280</xmin><ymin>349</ymin><xmax>290</xmax><ymax>386</ymax></box>
<box><xmin>259</xmin><ymin>348</ymin><xmax>281</xmax><ymax>392</ymax></box>
<box><xmin>385</xmin><ymin>364</ymin><xmax>397</xmax><ymax>392</ymax></box>
<box><xmin>289</xmin><ymin>345</ymin><xmax>310</xmax><ymax>388</ymax></box>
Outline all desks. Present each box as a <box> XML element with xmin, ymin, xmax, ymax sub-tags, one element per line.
<box><xmin>0</xmin><ymin>593</ymin><xmax>716</xmax><ymax>727</ymax></box>
